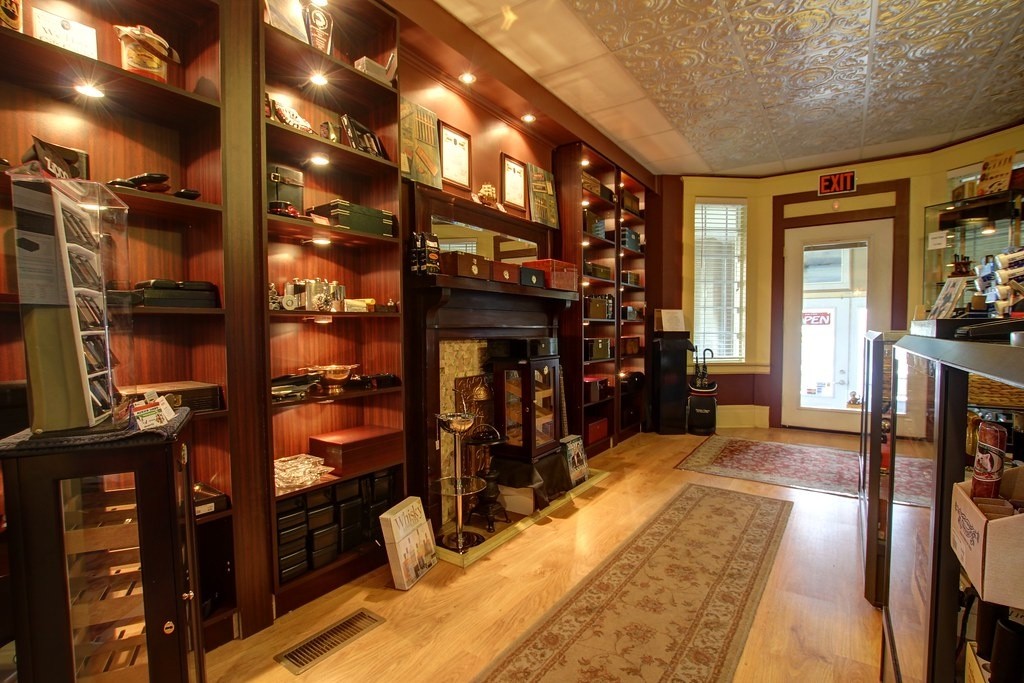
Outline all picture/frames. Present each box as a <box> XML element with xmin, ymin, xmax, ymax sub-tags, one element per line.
<box><xmin>926</xmin><ymin>277</ymin><xmax>967</xmax><ymax>320</ymax></box>
<box><xmin>341</xmin><ymin>114</ymin><xmax>385</xmax><ymax>161</ymax></box>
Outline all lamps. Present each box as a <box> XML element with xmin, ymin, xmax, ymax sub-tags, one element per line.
<box><xmin>72</xmin><ymin>77</ymin><xmax>106</xmax><ymax>100</ymax></box>
<box><xmin>309</xmin><ymin>152</ymin><xmax>330</xmax><ymax>169</ymax></box>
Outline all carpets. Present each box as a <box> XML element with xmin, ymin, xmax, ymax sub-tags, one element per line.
<box><xmin>470</xmin><ymin>482</ymin><xmax>793</xmax><ymax>683</ymax></box>
<box><xmin>893</xmin><ymin>454</ymin><xmax>933</xmax><ymax>507</ymax></box>
<box><xmin>673</xmin><ymin>431</ymin><xmax>860</xmax><ymax>503</ymax></box>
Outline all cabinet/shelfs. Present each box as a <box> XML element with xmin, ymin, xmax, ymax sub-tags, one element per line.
<box><xmin>0</xmin><ymin>0</ymin><xmax>410</xmax><ymax>654</ymax></box>
<box><xmin>401</xmin><ymin>174</ymin><xmax>580</xmax><ymax>513</ymax></box>
<box><xmin>488</xmin><ymin>355</ymin><xmax>562</xmax><ymax>464</ymax></box>
<box><xmin>654</xmin><ymin>330</ymin><xmax>696</xmax><ymax>435</ymax></box>
<box><xmin>0</xmin><ymin>406</ymin><xmax>208</xmax><ymax>683</ymax></box>
<box><xmin>5</xmin><ymin>161</ymin><xmax>135</xmax><ymax>438</ymax></box>
<box><xmin>853</xmin><ymin>330</ymin><xmax>911</xmax><ymax>613</ymax></box>
<box><xmin>879</xmin><ymin>334</ymin><xmax>1024</xmax><ymax>683</ymax></box>
<box><xmin>553</xmin><ymin>141</ymin><xmax>653</xmax><ymax>460</ymax></box>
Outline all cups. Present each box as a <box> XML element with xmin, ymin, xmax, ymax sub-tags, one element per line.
<box><xmin>990</xmin><ymin>620</ymin><xmax>1024</xmax><ymax>683</ymax></box>
<box><xmin>1010</xmin><ymin>331</ymin><xmax>1024</xmax><ymax>347</ymax></box>
<box><xmin>976</xmin><ymin>597</ymin><xmax>1009</xmax><ymax>662</ymax></box>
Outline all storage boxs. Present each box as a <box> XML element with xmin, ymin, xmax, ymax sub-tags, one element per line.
<box><xmin>264</xmin><ymin>161</ymin><xmax>394</xmax><ymax>241</ymax></box>
<box><xmin>965</xmin><ymin>641</ymin><xmax>992</xmax><ymax>683</ymax></box>
<box><xmin>581</xmin><ymin>168</ymin><xmax>640</xmax><ymax>321</ymax></box>
<box><xmin>277</xmin><ymin>468</ymin><xmax>396</xmax><ymax>587</ymax></box>
<box><xmin>950</xmin><ymin>466</ymin><xmax>1024</xmax><ymax>611</ymax></box>
<box><xmin>309</xmin><ymin>424</ymin><xmax>407</xmax><ymax>478</ymax></box>
<box><xmin>687</xmin><ymin>380</ymin><xmax>718</xmax><ymax>436</ymax></box>
<box><xmin>584</xmin><ymin>335</ymin><xmax>640</xmax><ymax>445</ymax></box>
<box><xmin>439</xmin><ymin>250</ymin><xmax>578</xmax><ymax>293</ymax></box>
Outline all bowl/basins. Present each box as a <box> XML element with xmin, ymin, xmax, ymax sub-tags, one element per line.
<box><xmin>307</xmin><ymin>364</ymin><xmax>359</xmax><ymax>389</ymax></box>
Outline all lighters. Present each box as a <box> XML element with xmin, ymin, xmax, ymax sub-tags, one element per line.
<box><xmin>60</xmin><ymin>208</ymin><xmax>119</xmax><ymax>411</ymax></box>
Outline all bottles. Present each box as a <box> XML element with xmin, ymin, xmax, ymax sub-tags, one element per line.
<box><xmin>387</xmin><ymin>299</ymin><xmax>395</xmax><ymax>313</ymax></box>
